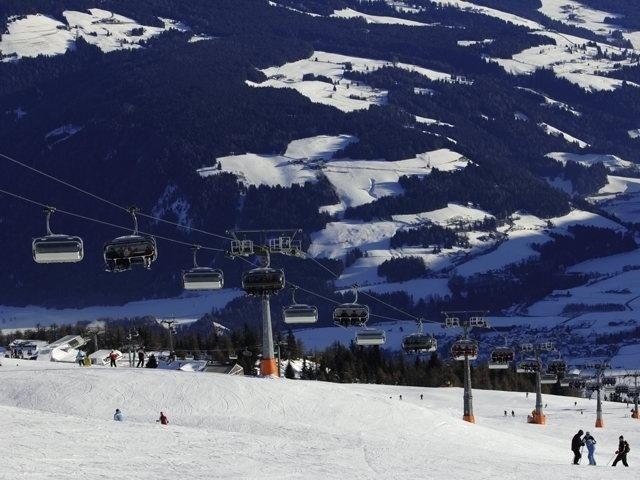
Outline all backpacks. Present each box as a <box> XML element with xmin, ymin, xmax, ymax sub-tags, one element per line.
<box><xmin>621</xmin><ymin>440</ymin><xmax>631</xmax><ymax>454</ymax></box>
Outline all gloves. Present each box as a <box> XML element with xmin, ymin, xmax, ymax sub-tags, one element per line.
<box><xmin>615</xmin><ymin>450</ymin><xmax>619</xmax><ymax>454</ymax></box>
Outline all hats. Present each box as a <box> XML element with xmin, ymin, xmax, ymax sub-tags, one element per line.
<box><xmin>586</xmin><ymin>431</ymin><xmax>590</xmax><ymax>435</ymax></box>
<box><xmin>619</xmin><ymin>435</ymin><xmax>624</xmax><ymax>439</ymax></box>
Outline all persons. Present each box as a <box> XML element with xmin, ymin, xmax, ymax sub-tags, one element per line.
<box><xmin>586</xmin><ymin>436</ymin><xmax>596</xmax><ymax>466</ymax></box>
<box><xmin>136</xmin><ymin>346</ymin><xmax>145</xmax><ymax>368</ymax></box>
<box><xmin>523</xmin><ymin>359</ymin><xmax>567</xmax><ymax>376</ymax></box>
<box><xmin>334</xmin><ymin>309</ymin><xmax>368</xmax><ymax>327</ymax></box>
<box><xmin>581</xmin><ymin>432</ymin><xmax>597</xmax><ymax>465</ymax></box>
<box><xmin>402</xmin><ymin>333</ymin><xmax>435</xmax><ymax>355</ymax></box>
<box><xmin>504</xmin><ymin>409</ymin><xmax>516</xmax><ymax>417</ymax></box>
<box><xmin>106</xmin><ymin>351</ymin><xmax>116</xmax><ymax>367</ymax></box>
<box><xmin>103</xmin><ymin>243</ymin><xmax>156</xmax><ymax>274</ymax></box>
<box><xmin>452</xmin><ymin>342</ymin><xmax>478</xmax><ymax>358</ymax></box>
<box><xmin>75</xmin><ymin>348</ymin><xmax>86</xmax><ymax>366</ymax></box>
<box><xmin>571</xmin><ymin>377</ymin><xmax>640</xmax><ymax>403</ymax></box>
<box><xmin>492</xmin><ymin>351</ymin><xmax>514</xmax><ymax>365</ymax></box>
<box><xmin>571</xmin><ymin>429</ymin><xmax>585</xmax><ymax>465</ymax></box>
<box><xmin>612</xmin><ymin>436</ymin><xmax>629</xmax><ymax>467</ymax></box>
<box><xmin>243</xmin><ymin>269</ymin><xmax>281</xmax><ymax>302</ymax></box>
<box><xmin>156</xmin><ymin>411</ymin><xmax>170</xmax><ymax>425</ymax></box>
<box><xmin>145</xmin><ymin>353</ymin><xmax>158</xmax><ymax>368</ymax></box>
<box><xmin>114</xmin><ymin>408</ymin><xmax>123</xmax><ymax>421</ymax></box>
